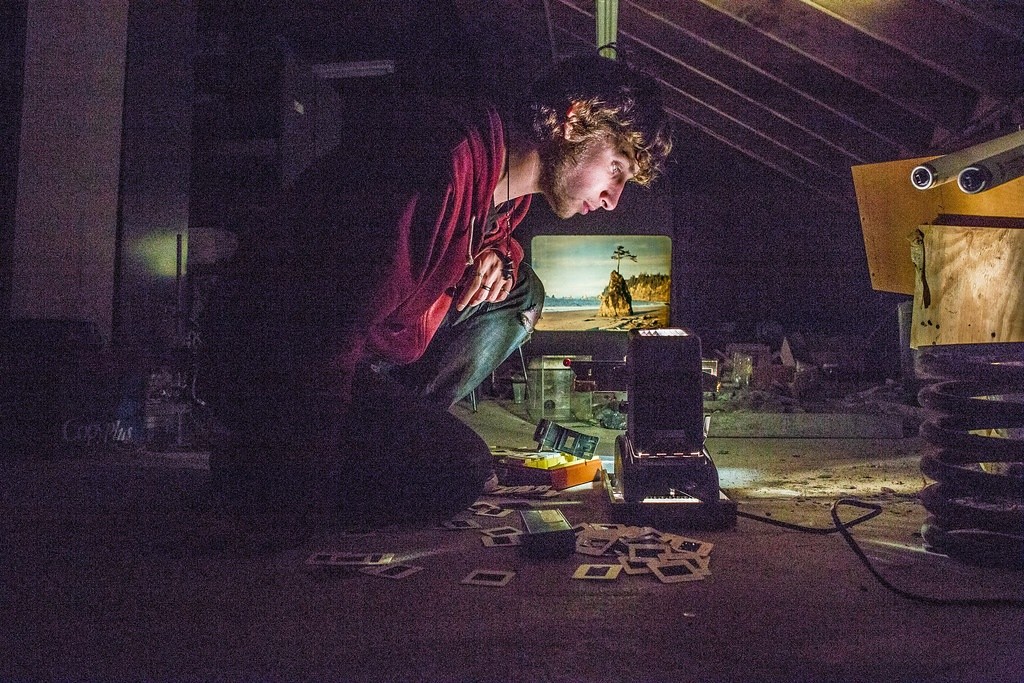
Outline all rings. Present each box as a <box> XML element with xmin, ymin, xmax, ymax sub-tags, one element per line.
<box><xmin>500</xmin><ymin>287</ymin><xmax>510</xmax><ymax>296</ymax></box>
<box><xmin>473</xmin><ymin>270</ymin><xmax>483</xmax><ymax>278</ymax></box>
<box><xmin>480</xmin><ymin>284</ymin><xmax>491</xmax><ymax>292</ymax></box>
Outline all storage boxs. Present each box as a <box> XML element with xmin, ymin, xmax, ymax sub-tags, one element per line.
<box><xmin>520</xmin><ymin>509</ymin><xmax>575</xmax><ymax>555</ymax></box>
<box><xmin>492</xmin><ymin>459</ymin><xmax>600</xmax><ymax>490</ymax></box>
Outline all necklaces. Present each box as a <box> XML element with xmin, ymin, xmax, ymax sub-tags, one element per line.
<box><xmin>500</xmin><ymin>115</ymin><xmax>516</xmax><ymax>283</ymax></box>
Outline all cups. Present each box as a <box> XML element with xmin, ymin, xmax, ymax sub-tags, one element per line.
<box><xmin>513</xmin><ymin>383</ymin><xmax>526</xmax><ymax>404</ymax></box>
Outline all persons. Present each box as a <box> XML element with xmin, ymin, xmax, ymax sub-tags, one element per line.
<box><xmin>189</xmin><ymin>39</ymin><xmax>678</xmax><ymax>556</ymax></box>
